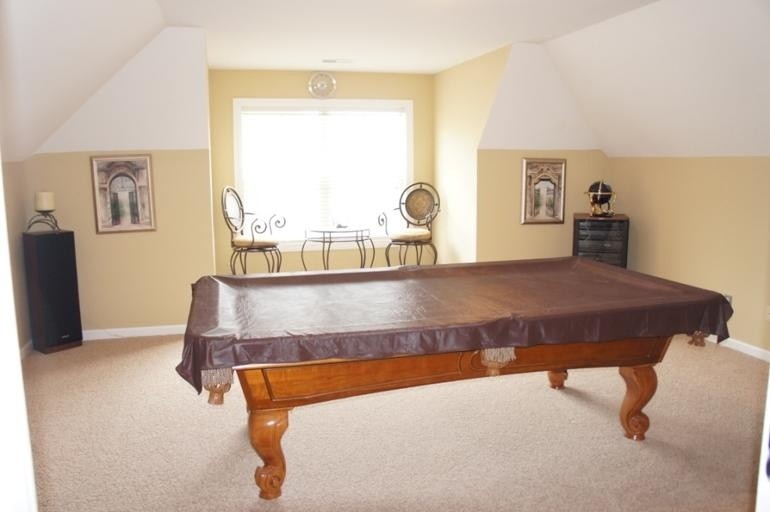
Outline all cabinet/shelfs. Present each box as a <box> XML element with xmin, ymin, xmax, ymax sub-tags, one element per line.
<box><xmin>570</xmin><ymin>210</ymin><xmax>631</xmax><ymax>267</ymax></box>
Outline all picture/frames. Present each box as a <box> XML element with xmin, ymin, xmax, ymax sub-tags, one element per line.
<box><xmin>89</xmin><ymin>153</ymin><xmax>157</xmax><ymax>236</ymax></box>
<box><xmin>520</xmin><ymin>156</ymin><xmax>566</xmax><ymax>227</ymax></box>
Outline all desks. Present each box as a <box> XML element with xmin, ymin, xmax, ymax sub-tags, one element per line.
<box><xmin>185</xmin><ymin>252</ymin><xmax>728</xmax><ymax>499</ymax></box>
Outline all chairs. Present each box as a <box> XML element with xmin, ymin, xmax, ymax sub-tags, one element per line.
<box><xmin>218</xmin><ymin>180</ymin><xmax>442</xmax><ymax>274</ymax></box>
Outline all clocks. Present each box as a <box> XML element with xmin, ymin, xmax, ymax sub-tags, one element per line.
<box><xmin>306</xmin><ymin>70</ymin><xmax>337</xmax><ymax>99</ymax></box>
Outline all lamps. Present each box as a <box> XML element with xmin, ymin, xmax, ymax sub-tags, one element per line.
<box><xmin>23</xmin><ymin>189</ymin><xmax>59</xmax><ymax>232</ymax></box>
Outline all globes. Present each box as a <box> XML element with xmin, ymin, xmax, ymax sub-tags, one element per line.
<box><xmin>583</xmin><ymin>177</ymin><xmax>616</xmax><ymax>217</ymax></box>
